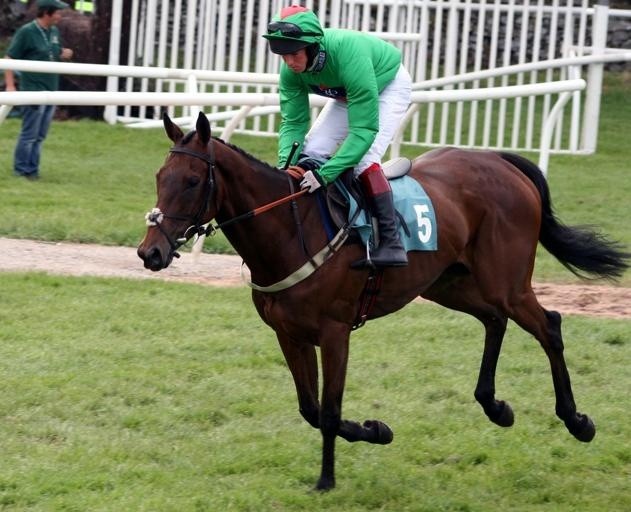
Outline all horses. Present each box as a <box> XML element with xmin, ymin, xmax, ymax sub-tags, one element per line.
<box><xmin>137</xmin><ymin>111</ymin><xmax>631</xmax><ymax>495</ymax></box>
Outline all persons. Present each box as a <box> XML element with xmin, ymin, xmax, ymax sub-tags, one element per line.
<box><xmin>3</xmin><ymin>0</ymin><xmax>74</xmax><ymax>183</ymax></box>
<box><xmin>261</xmin><ymin>4</ymin><xmax>413</xmax><ymax>269</ymax></box>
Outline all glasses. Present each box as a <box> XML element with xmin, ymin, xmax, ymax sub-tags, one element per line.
<box><xmin>267</xmin><ymin>21</ymin><xmax>320</xmax><ymax>37</ymax></box>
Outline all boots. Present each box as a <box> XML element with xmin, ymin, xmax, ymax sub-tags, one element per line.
<box><xmin>349</xmin><ymin>163</ymin><xmax>408</xmax><ymax>268</ymax></box>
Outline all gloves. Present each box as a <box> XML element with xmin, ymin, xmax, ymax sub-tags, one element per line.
<box><xmin>299</xmin><ymin>170</ymin><xmax>323</xmax><ymax>193</ymax></box>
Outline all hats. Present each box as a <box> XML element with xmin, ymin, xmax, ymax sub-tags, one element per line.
<box><xmin>38</xmin><ymin>0</ymin><xmax>69</xmax><ymax>9</ymax></box>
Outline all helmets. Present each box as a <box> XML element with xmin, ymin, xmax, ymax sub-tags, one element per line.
<box><xmin>270</xmin><ymin>38</ymin><xmax>310</xmax><ymax>54</ymax></box>
